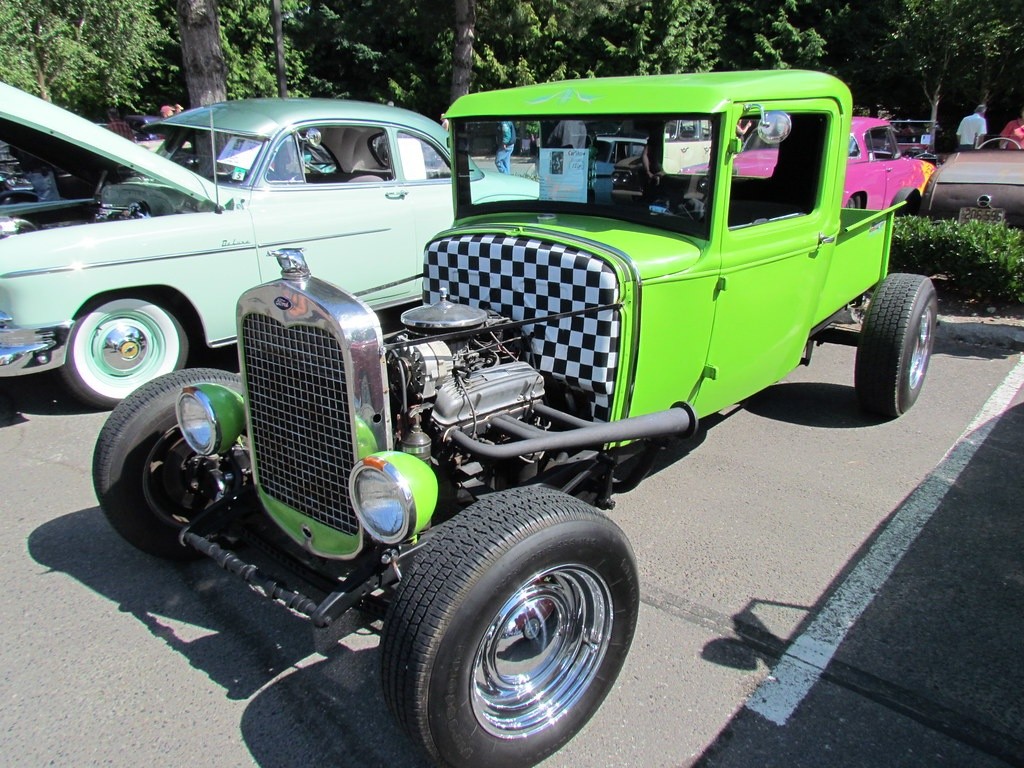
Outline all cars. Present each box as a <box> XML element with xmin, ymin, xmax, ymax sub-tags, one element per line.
<box><xmin>678</xmin><ymin>115</ymin><xmax>935</xmax><ymax>210</ymax></box>
<box><xmin>92</xmin><ymin>66</ymin><xmax>938</xmax><ymax>767</ymax></box>
<box><xmin>889</xmin><ymin>133</ymin><xmax>1024</xmax><ymax>230</ymax></box>
<box><xmin>1</xmin><ymin>80</ymin><xmax>540</xmax><ymax>408</ymax></box>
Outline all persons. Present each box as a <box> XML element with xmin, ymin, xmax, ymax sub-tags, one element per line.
<box><xmin>998</xmin><ymin>109</ymin><xmax>1024</xmax><ymax>150</ymax></box>
<box><xmin>493</xmin><ymin>121</ymin><xmax>516</xmax><ymax>175</ymax></box>
<box><xmin>637</xmin><ymin>130</ymin><xmax>690</xmax><ymax>207</ymax></box>
<box><xmin>173</xmin><ymin>103</ymin><xmax>182</xmax><ymax>116</ymax></box>
<box><xmin>736</xmin><ymin>117</ymin><xmax>752</xmax><ymax>146</ymax></box>
<box><xmin>106</xmin><ymin>108</ymin><xmax>134</xmax><ymax>142</ymax></box>
<box><xmin>158</xmin><ymin>106</ymin><xmax>174</xmax><ymax>140</ymax></box>
<box><xmin>954</xmin><ymin>104</ymin><xmax>989</xmax><ymax>151</ymax></box>
<box><xmin>439</xmin><ymin>113</ymin><xmax>449</xmax><ymax>131</ymax></box>
<box><xmin>546</xmin><ymin>120</ymin><xmax>586</xmax><ymax>149</ymax></box>
